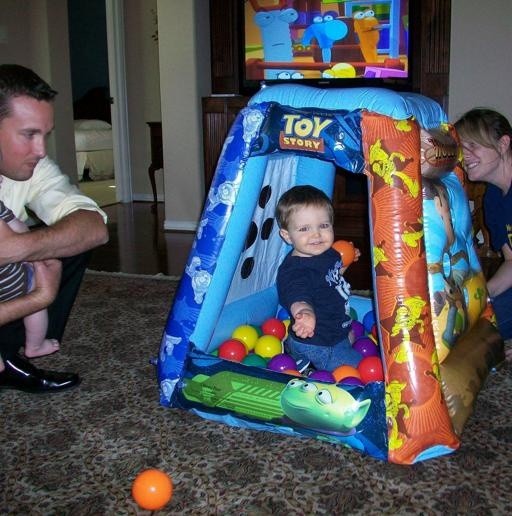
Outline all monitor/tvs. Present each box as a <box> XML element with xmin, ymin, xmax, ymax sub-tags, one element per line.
<box><xmin>234</xmin><ymin>0</ymin><xmax>419</xmax><ymax>94</ymax></box>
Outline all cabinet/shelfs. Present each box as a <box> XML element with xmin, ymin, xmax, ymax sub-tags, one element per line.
<box><xmin>202</xmin><ymin>96</ymin><xmax>249</xmax><ymax>208</ymax></box>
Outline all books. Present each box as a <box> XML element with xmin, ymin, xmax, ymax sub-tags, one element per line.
<box><xmin>210</xmin><ymin>93</ymin><xmax>242</xmax><ymax>97</ymax></box>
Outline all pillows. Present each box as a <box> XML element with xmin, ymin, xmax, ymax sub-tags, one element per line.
<box><xmin>72</xmin><ymin>119</ymin><xmax>112</xmax><ymax>131</ymax></box>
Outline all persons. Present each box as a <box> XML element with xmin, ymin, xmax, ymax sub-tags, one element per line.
<box><xmin>453</xmin><ymin>108</ymin><xmax>512</xmax><ymax>341</ymax></box>
<box><xmin>0</xmin><ymin>62</ymin><xmax>111</xmax><ymax>394</ymax></box>
<box><xmin>0</xmin><ymin>173</ymin><xmax>61</xmax><ymax>372</ymax></box>
<box><xmin>275</xmin><ymin>183</ymin><xmax>366</xmax><ymax>372</ymax></box>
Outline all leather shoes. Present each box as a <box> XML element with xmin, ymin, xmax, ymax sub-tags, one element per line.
<box><xmin>0</xmin><ymin>356</ymin><xmax>85</xmax><ymax>395</ymax></box>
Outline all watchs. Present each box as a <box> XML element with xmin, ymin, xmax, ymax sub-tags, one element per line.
<box><xmin>484</xmin><ymin>286</ymin><xmax>494</xmax><ymax>305</ymax></box>
